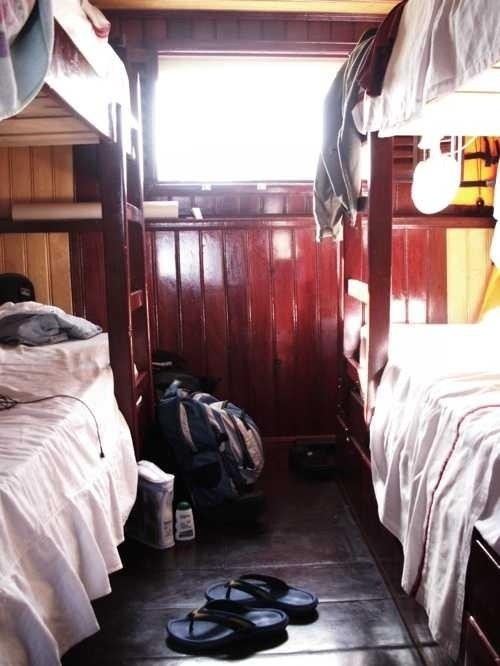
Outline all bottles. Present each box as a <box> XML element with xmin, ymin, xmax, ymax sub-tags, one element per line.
<box><xmin>175</xmin><ymin>502</ymin><xmax>196</xmax><ymax>541</ymax></box>
<box><xmin>358</xmin><ymin>179</ymin><xmax>370</xmax><ymax>210</ymax></box>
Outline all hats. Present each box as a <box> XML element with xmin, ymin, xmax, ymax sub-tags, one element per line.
<box><xmin>0</xmin><ymin>273</ymin><xmax>34</xmax><ymax>303</ymax></box>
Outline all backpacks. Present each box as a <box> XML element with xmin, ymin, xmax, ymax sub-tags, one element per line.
<box><xmin>157</xmin><ymin>378</ymin><xmax>265</xmax><ymax>511</ymax></box>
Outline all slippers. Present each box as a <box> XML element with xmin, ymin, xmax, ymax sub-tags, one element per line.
<box><xmin>205</xmin><ymin>573</ymin><xmax>317</xmax><ymax>613</ymax></box>
<box><xmin>167</xmin><ymin>599</ymin><xmax>287</xmax><ymax>649</ymax></box>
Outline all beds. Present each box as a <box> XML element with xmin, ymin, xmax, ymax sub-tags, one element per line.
<box><xmin>1</xmin><ymin>1</ymin><xmax>152</xmax><ymax>666</ymax></box>
<box><xmin>314</xmin><ymin>0</ymin><xmax>499</xmax><ymax>665</ymax></box>
